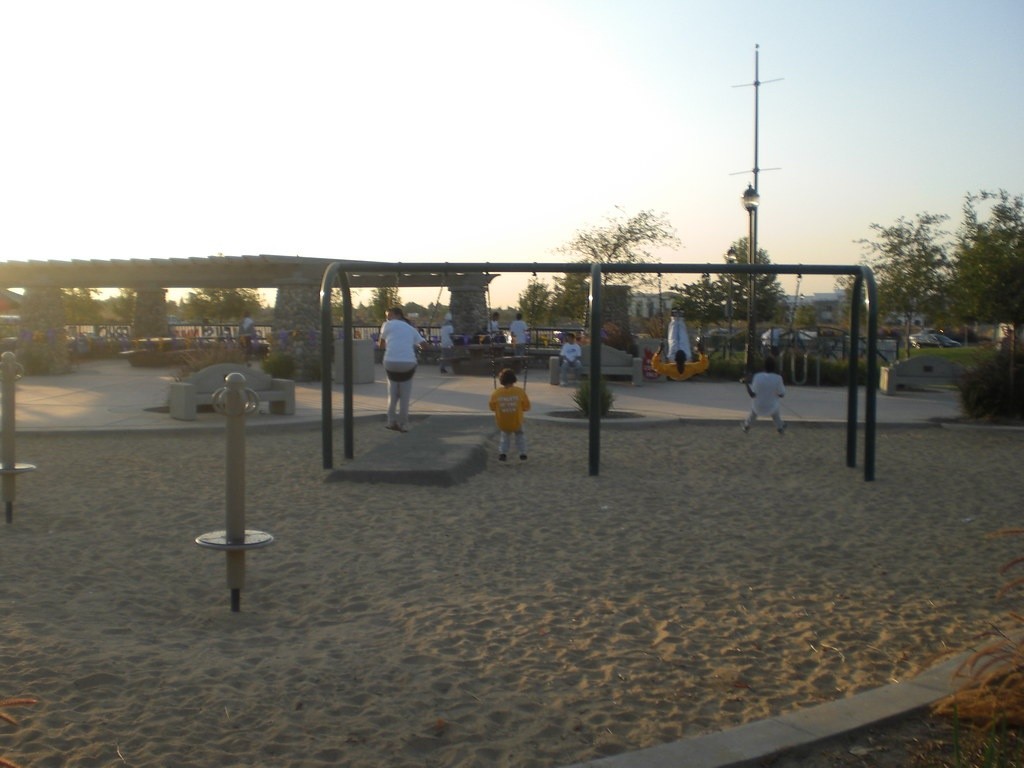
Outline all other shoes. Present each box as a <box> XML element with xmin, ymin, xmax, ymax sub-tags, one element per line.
<box><xmin>740</xmin><ymin>422</ymin><xmax>748</xmax><ymax>432</ymax></box>
<box><xmin>521</xmin><ymin>455</ymin><xmax>527</xmax><ymax>460</ymax></box>
<box><xmin>387</xmin><ymin>424</ymin><xmax>400</xmax><ymax>431</ymax></box>
<box><xmin>778</xmin><ymin>423</ymin><xmax>787</xmax><ymax>432</ymax></box>
<box><xmin>499</xmin><ymin>454</ymin><xmax>506</xmax><ymax>460</ymax></box>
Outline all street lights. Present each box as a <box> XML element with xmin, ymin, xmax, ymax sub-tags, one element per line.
<box><xmin>742</xmin><ymin>184</ymin><xmax>760</xmax><ymax>381</ymax></box>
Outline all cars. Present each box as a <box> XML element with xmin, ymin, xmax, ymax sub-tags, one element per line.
<box><xmin>762</xmin><ymin>328</ymin><xmax>817</xmax><ymax>350</ymax></box>
<box><xmin>910</xmin><ymin>328</ymin><xmax>943</xmax><ymax>340</ymax></box>
<box><xmin>912</xmin><ymin>333</ymin><xmax>961</xmax><ymax>351</ymax></box>
<box><xmin>695</xmin><ymin>329</ymin><xmax>739</xmax><ymax>348</ymax></box>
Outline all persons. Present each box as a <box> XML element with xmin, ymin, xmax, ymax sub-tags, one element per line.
<box><xmin>650</xmin><ymin>307</ymin><xmax>710</xmax><ymax>382</ymax></box>
<box><xmin>488</xmin><ymin>312</ymin><xmax>500</xmax><ymax>334</ymax></box>
<box><xmin>379</xmin><ymin>307</ymin><xmax>430</xmax><ymax>433</ymax></box>
<box><xmin>489</xmin><ymin>368</ymin><xmax>531</xmax><ymax>461</ymax></box>
<box><xmin>238</xmin><ymin>311</ymin><xmax>258</xmax><ymax>343</ymax></box>
<box><xmin>740</xmin><ymin>359</ymin><xmax>788</xmax><ymax>435</ymax></box>
<box><xmin>510</xmin><ymin>313</ymin><xmax>528</xmax><ymax>344</ymax></box>
<box><xmin>441</xmin><ymin>313</ymin><xmax>455</xmax><ymax>373</ymax></box>
<box><xmin>559</xmin><ymin>333</ymin><xmax>583</xmax><ymax>387</ymax></box>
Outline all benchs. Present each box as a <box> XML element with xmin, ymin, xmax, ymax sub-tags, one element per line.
<box><xmin>168</xmin><ymin>363</ymin><xmax>295</xmax><ymax>420</ymax></box>
<box><xmin>164</xmin><ymin>348</ymin><xmax>200</xmax><ymax>363</ymax></box>
<box><xmin>119</xmin><ymin>348</ymin><xmax>146</xmax><ymax>367</ymax></box>
<box><xmin>468</xmin><ymin>343</ymin><xmax>534</xmax><ymax>377</ymax></box>
<box><xmin>549</xmin><ymin>344</ymin><xmax>643</xmax><ymax>386</ymax></box>
<box><xmin>879</xmin><ymin>355</ymin><xmax>964</xmax><ymax>395</ymax></box>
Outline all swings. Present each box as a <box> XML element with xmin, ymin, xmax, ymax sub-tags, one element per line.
<box><xmin>481</xmin><ymin>272</ymin><xmax>538</xmax><ymax>411</ymax></box>
<box><xmin>743</xmin><ymin>271</ymin><xmax>802</xmax><ymax>400</ymax></box>
<box><xmin>384</xmin><ymin>271</ymin><xmax>448</xmax><ymax>383</ymax></box>
<box><xmin>656</xmin><ymin>273</ymin><xmax>710</xmax><ymax>382</ymax></box>
